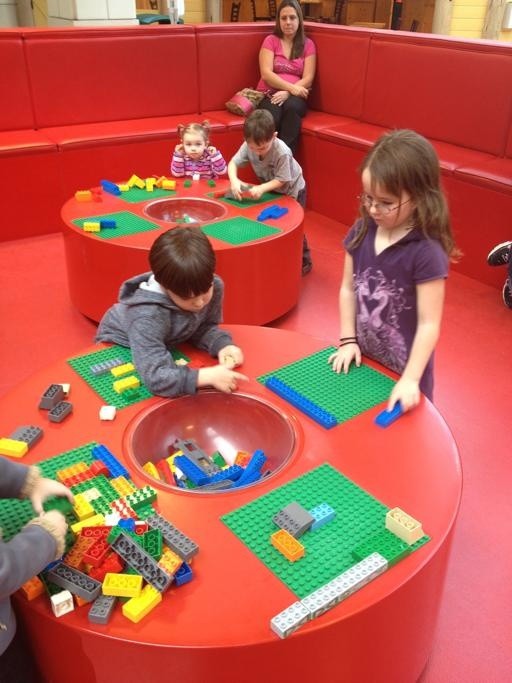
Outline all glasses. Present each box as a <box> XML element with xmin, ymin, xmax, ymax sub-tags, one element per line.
<box><xmin>355</xmin><ymin>191</ymin><xmax>412</xmax><ymax>215</ymax></box>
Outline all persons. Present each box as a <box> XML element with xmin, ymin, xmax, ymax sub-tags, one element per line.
<box><xmin>94</xmin><ymin>225</ymin><xmax>250</xmax><ymax>398</ymax></box>
<box><xmin>228</xmin><ymin>110</ymin><xmax>312</xmax><ymax>277</ymax></box>
<box><xmin>486</xmin><ymin>240</ymin><xmax>512</xmax><ymax>309</ymax></box>
<box><xmin>171</xmin><ymin>120</ymin><xmax>228</xmax><ymax>179</ymax></box>
<box><xmin>0</xmin><ymin>456</ymin><xmax>76</xmax><ymax>683</ymax></box>
<box><xmin>256</xmin><ymin>0</ymin><xmax>317</xmax><ymax>157</ymax></box>
<box><xmin>327</xmin><ymin>127</ymin><xmax>464</xmax><ymax>414</ymax></box>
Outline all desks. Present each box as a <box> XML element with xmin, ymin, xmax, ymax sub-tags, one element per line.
<box><xmin>0</xmin><ymin>323</ymin><xmax>461</xmax><ymax>682</ymax></box>
<box><xmin>61</xmin><ymin>176</ymin><xmax>305</xmax><ymax>329</ymax></box>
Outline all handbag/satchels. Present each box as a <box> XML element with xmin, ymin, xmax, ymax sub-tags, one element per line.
<box><xmin>226</xmin><ymin>87</ymin><xmax>272</xmax><ymax>117</ymax></box>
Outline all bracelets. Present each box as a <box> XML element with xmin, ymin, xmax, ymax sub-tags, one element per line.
<box><xmin>340</xmin><ymin>337</ymin><xmax>357</xmax><ymax>341</ymax></box>
<box><xmin>339</xmin><ymin>342</ymin><xmax>358</xmax><ymax>347</ymax></box>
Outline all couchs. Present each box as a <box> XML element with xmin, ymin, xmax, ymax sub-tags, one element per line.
<box><xmin>1</xmin><ymin>19</ymin><xmax>512</xmax><ymax>302</ymax></box>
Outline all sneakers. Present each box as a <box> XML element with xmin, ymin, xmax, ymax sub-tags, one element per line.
<box><xmin>302</xmin><ymin>264</ymin><xmax>311</xmax><ymax>274</ymax></box>
<box><xmin>502</xmin><ymin>279</ymin><xmax>512</xmax><ymax>308</ymax></box>
<box><xmin>487</xmin><ymin>241</ymin><xmax>512</xmax><ymax>266</ymax></box>
